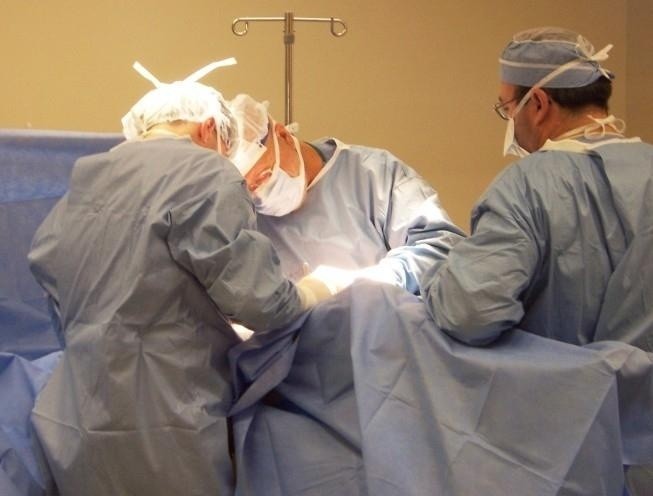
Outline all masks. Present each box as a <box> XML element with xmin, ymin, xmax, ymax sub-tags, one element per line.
<box><xmin>253</xmin><ymin>134</ymin><xmax>306</xmax><ymax>217</ymax></box>
<box><xmin>503</xmin><ymin>92</ymin><xmax>540</xmax><ymax>156</ymax></box>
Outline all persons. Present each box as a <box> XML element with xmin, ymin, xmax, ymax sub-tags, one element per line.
<box><xmin>24</xmin><ymin>55</ymin><xmax>353</xmax><ymax>496</ymax></box>
<box><xmin>218</xmin><ymin>91</ymin><xmax>468</xmax><ymax>295</ymax></box>
<box><xmin>424</xmin><ymin>23</ymin><xmax>650</xmax><ymax>354</ymax></box>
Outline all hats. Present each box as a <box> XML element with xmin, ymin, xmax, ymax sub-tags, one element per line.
<box><xmin>499</xmin><ymin>27</ymin><xmax>614</xmax><ymax>88</ymax></box>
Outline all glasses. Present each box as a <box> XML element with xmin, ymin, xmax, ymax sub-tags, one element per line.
<box><xmin>494</xmin><ymin>98</ymin><xmax>518</xmax><ymax>121</ymax></box>
